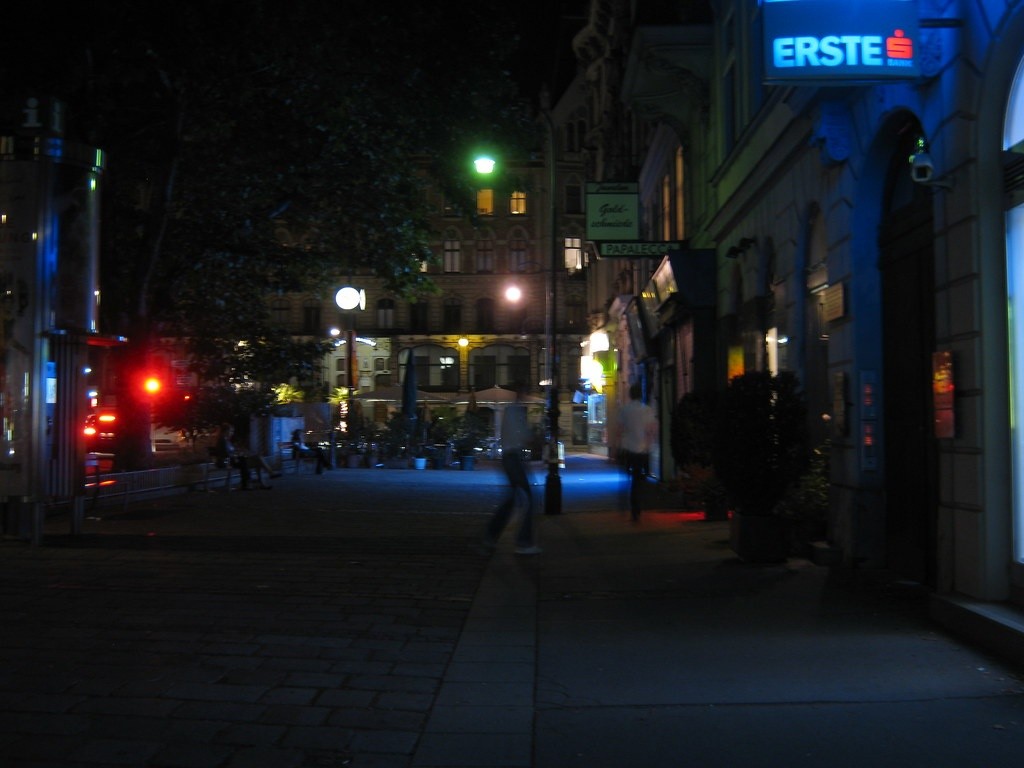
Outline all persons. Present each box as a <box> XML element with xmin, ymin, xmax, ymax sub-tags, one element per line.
<box><xmin>617</xmin><ymin>384</ymin><xmax>660</xmax><ymax>522</ymax></box>
<box><xmin>215</xmin><ymin>422</ymin><xmax>282</xmax><ymax>489</ymax></box>
<box><xmin>468</xmin><ymin>377</ymin><xmax>545</xmax><ymax>556</ymax></box>
<box><xmin>293</xmin><ymin>431</ymin><xmax>334</xmax><ymax>474</ymax></box>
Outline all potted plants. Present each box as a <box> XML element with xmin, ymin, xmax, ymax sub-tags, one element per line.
<box><xmin>667</xmin><ymin>371</ymin><xmax>812</xmax><ymax>567</ymax></box>
<box><xmin>340</xmin><ymin>404</ymin><xmax>482</xmax><ymax>470</ymax></box>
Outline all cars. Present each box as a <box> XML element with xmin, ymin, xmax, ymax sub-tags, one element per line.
<box><xmin>81</xmin><ymin>389</ymin><xmax>124</xmax><ymax>454</ymax></box>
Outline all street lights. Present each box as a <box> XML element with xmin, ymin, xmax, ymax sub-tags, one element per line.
<box><xmin>335</xmin><ymin>285</ymin><xmax>368</xmax><ymax>405</ymax></box>
<box><xmin>463</xmin><ymin>103</ymin><xmax>562</xmax><ymax>517</ymax></box>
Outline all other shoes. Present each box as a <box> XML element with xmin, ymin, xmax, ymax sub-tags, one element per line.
<box><xmin>483</xmin><ymin>530</ymin><xmax>502</xmax><ymax>551</ymax></box>
<box><xmin>269</xmin><ymin>472</ymin><xmax>282</xmax><ymax>479</ymax></box>
<box><xmin>240</xmin><ymin>486</ymin><xmax>253</xmax><ymax>491</ymax></box>
<box><xmin>262</xmin><ymin>484</ymin><xmax>274</xmax><ymax>490</ymax></box>
<box><xmin>514</xmin><ymin>546</ymin><xmax>542</xmax><ymax>556</ymax></box>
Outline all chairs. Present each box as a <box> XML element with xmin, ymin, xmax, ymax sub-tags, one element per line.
<box><xmin>293</xmin><ymin>446</ymin><xmax>320</xmax><ymax>475</ymax></box>
<box><xmin>204</xmin><ymin>448</ymin><xmax>233</xmax><ymax>491</ymax></box>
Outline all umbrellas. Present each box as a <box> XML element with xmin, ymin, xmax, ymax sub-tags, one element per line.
<box><xmin>353</xmin><ymin>383</ymin><xmax>545</xmax><ymax>409</ymax></box>
<box><xmin>402</xmin><ymin>349</ymin><xmax>416</xmax><ymax>422</ymax></box>
